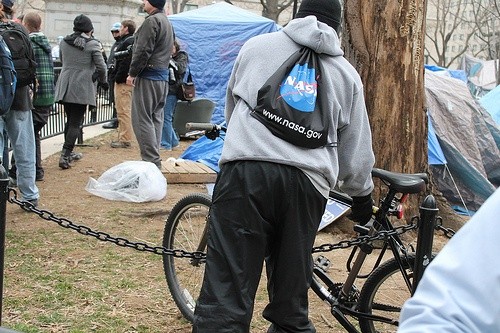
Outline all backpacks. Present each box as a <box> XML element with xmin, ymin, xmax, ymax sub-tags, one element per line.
<box><xmin>0</xmin><ymin>22</ymin><xmax>38</xmax><ymax>100</ymax></box>
<box><xmin>243</xmin><ymin>46</ymin><xmax>348</xmax><ymax>149</ymax></box>
<box><xmin>0</xmin><ymin>35</ymin><xmax>18</xmax><ymax>123</ymax></box>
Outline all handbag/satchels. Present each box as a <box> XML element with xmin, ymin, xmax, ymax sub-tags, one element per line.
<box><xmin>176</xmin><ymin>82</ymin><xmax>196</xmax><ymax>102</ymax></box>
<box><xmin>85</xmin><ymin>159</ymin><xmax>168</xmax><ymax>204</ymax></box>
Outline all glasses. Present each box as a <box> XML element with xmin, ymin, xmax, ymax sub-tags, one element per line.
<box><xmin>111</xmin><ymin>30</ymin><xmax>119</xmax><ymax>34</ymax></box>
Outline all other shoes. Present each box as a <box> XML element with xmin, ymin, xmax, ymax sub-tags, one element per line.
<box><xmin>35</xmin><ymin>176</ymin><xmax>44</xmax><ymax>181</ymax></box>
<box><xmin>20</xmin><ymin>199</ymin><xmax>39</xmax><ymax>207</ymax></box>
<box><xmin>110</xmin><ymin>139</ymin><xmax>131</xmax><ymax>148</ymax></box>
<box><xmin>160</xmin><ymin>143</ymin><xmax>180</xmax><ymax>152</ymax></box>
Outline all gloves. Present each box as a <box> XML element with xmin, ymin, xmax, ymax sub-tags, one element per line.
<box><xmin>100</xmin><ymin>83</ymin><xmax>109</xmax><ymax>91</ymax></box>
<box><xmin>115</xmin><ymin>73</ymin><xmax>126</xmax><ymax>85</ymax></box>
<box><xmin>346</xmin><ymin>192</ymin><xmax>373</xmax><ymax>226</ymax></box>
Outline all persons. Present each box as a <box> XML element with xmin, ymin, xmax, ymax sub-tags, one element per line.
<box><xmin>89</xmin><ymin>30</ymin><xmax>106</xmax><ymax>121</ymax></box>
<box><xmin>126</xmin><ymin>0</ymin><xmax>175</xmax><ymax>169</ymax></box>
<box><xmin>0</xmin><ymin>0</ymin><xmax>55</xmax><ymax>210</ymax></box>
<box><xmin>102</xmin><ymin>22</ymin><xmax>122</xmax><ymax>129</ymax></box>
<box><xmin>54</xmin><ymin>14</ymin><xmax>109</xmax><ymax>169</ymax></box>
<box><xmin>396</xmin><ymin>187</ymin><xmax>500</xmax><ymax>333</ymax></box>
<box><xmin>52</xmin><ymin>35</ymin><xmax>63</xmax><ymax>57</ymax></box>
<box><xmin>191</xmin><ymin>0</ymin><xmax>375</xmax><ymax>333</ymax></box>
<box><xmin>159</xmin><ymin>40</ymin><xmax>189</xmax><ymax>152</ymax></box>
<box><xmin>111</xmin><ymin>20</ymin><xmax>135</xmax><ymax>148</ymax></box>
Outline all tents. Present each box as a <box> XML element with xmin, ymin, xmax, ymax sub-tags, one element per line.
<box><xmin>424</xmin><ymin>55</ymin><xmax>500</xmax><ymax>216</ymax></box>
<box><xmin>167</xmin><ymin>1</ymin><xmax>284</xmax><ymax>132</ymax></box>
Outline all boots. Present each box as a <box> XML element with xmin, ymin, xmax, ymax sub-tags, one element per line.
<box><xmin>58</xmin><ymin>148</ymin><xmax>82</xmax><ymax>169</ymax></box>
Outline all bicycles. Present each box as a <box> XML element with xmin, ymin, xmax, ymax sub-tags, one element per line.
<box><xmin>162</xmin><ymin>121</ymin><xmax>437</xmax><ymax>333</ymax></box>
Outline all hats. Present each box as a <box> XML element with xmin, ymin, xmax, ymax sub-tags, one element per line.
<box><xmin>296</xmin><ymin>0</ymin><xmax>342</xmax><ymax>31</ymax></box>
<box><xmin>111</xmin><ymin>23</ymin><xmax>121</xmax><ymax>31</ymax></box>
<box><xmin>148</xmin><ymin>0</ymin><xmax>166</xmax><ymax>10</ymax></box>
<box><xmin>73</xmin><ymin>14</ymin><xmax>93</xmax><ymax>33</ymax></box>
